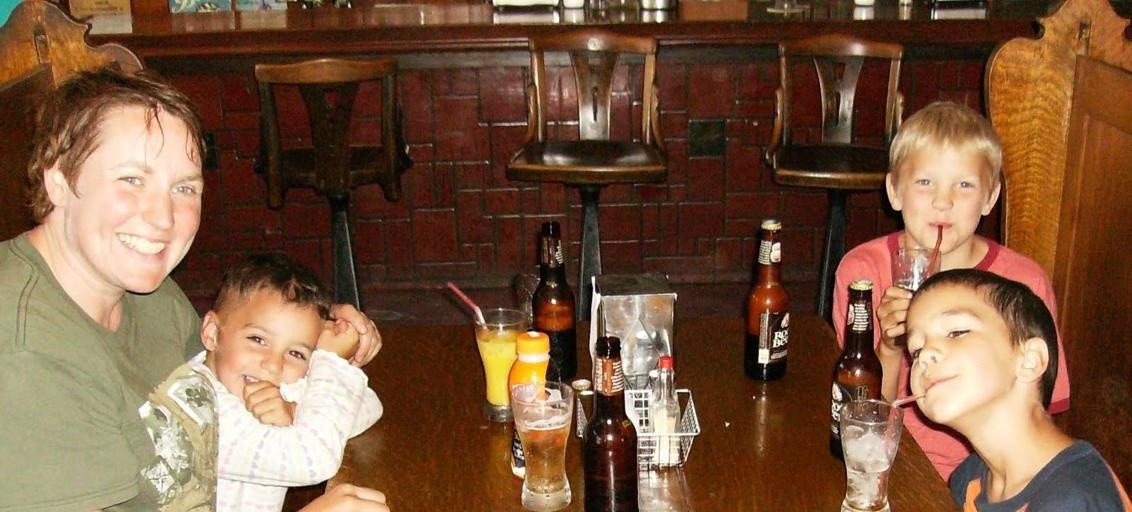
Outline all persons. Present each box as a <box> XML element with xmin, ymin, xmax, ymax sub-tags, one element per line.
<box><xmin>832</xmin><ymin>100</ymin><xmax>1073</xmax><ymax>485</ymax></box>
<box><xmin>196</xmin><ymin>251</ymin><xmax>385</xmax><ymax>512</ymax></box>
<box><xmin>0</xmin><ymin>66</ymin><xmax>386</xmax><ymax>511</ymax></box>
<box><xmin>904</xmin><ymin>268</ymin><xmax>1131</xmax><ymax>511</ymax></box>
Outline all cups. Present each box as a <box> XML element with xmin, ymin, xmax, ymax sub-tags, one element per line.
<box><xmin>889</xmin><ymin>246</ymin><xmax>939</xmax><ymax>346</ymax></box>
<box><xmin>508</xmin><ymin>380</ymin><xmax>573</xmax><ymax>511</ymax></box>
<box><xmin>512</xmin><ymin>273</ymin><xmax>541</xmax><ymax>330</ymax></box>
<box><xmin>471</xmin><ymin>307</ymin><xmax>526</xmax><ymax>423</ymax></box>
<box><xmin>835</xmin><ymin>399</ymin><xmax>905</xmax><ymax>511</ymax></box>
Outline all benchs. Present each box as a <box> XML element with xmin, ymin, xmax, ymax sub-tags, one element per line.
<box><xmin>0</xmin><ymin>0</ymin><xmax>149</xmax><ymax>249</ymax></box>
<box><xmin>974</xmin><ymin>1</ymin><xmax>1131</xmax><ymax>509</ymax></box>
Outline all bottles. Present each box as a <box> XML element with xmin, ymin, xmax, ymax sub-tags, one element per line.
<box><xmin>507</xmin><ymin>329</ymin><xmax>563</xmax><ymax>480</ymax></box>
<box><xmin>571</xmin><ymin>378</ymin><xmax>593</xmax><ymax>439</ymax></box>
<box><xmin>649</xmin><ymin>355</ymin><xmax>684</xmax><ymax>472</ymax></box>
<box><xmin>534</xmin><ymin>221</ymin><xmax>577</xmax><ymax>383</ymax></box>
<box><xmin>742</xmin><ymin>217</ymin><xmax>793</xmax><ymax>383</ymax></box>
<box><xmin>829</xmin><ymin>277</ymin><xmax>884</xmax><ymax>461</ymax></box>
<box><xmin>581</xmin><ymin>334</ymin><xmax>639</xmax><ymax>509</ymax></box>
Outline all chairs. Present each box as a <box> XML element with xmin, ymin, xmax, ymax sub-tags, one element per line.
<box><xmin>760</xmin><ymin>32</ymin><xmax>925</xmax><ymax>320</ymax></box>
<box><xmin>246</xmin><ymin>55</ymin><xmax>415</xmax><ymax>313</ymax></box>
<box><xmin>501</xmin><ymin>26</ymin><xmax>678</xmax><ymax>319</ymax></box>
